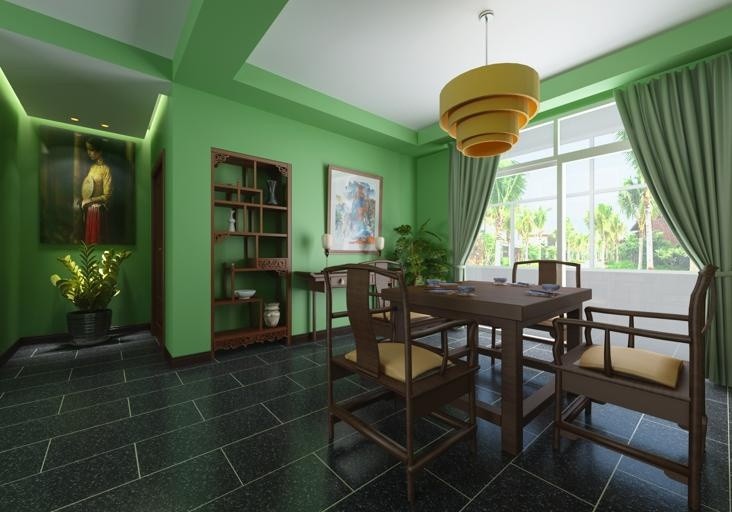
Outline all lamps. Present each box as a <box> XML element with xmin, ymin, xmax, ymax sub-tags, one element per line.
<box><xmin>323</xmin><ymin>234</ymin><xmax>331</xmax><ymax>268</ymax></box>
<box><xmin>440</xmin><ymin>10</ymin><xmax>541</xmax><ymax>158</ymax></box>
<box><xmin>376</xmin><ymin>237</ymin><xmax>384</xmax><ymax>261</ymax></box>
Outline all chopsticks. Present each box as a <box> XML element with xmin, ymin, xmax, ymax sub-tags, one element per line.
<box><xmin>423</xmin><ymin>287</ymin><xmax>457</xmax><ymax>291</ymax></box>
<box><xmin>530</xmin><ymin>288</ymin><xmax>558</xmax><ymax>296</ymax></box>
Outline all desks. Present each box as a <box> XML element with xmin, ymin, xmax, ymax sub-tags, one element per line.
<box><xmin>294</xmin><ymin>269</ymin><xmax>406</xmax><ymax>342</ymax></box>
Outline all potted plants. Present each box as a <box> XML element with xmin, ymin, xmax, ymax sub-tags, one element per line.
<box><xmin>51</xmin><ymin>241</ymin><xmax>132</xmax><ymax>347</ymax></box>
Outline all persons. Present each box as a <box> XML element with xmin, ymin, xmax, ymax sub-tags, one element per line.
<box><xmin>80</xmin><ymin>136</ymin><xmax>113</xmax><ymax>244</ymax></box>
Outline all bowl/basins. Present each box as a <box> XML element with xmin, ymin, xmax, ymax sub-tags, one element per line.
<box><xmin>494</xmin><ymin>277</ymin><xmax>507</xmax><ymax>284</ymax></box>
<box><xmin>234</xmin><ymin>289</ymin><xmax>257</xmax><ymax>300</ymax></box>
<box><xmin>426</xmin><ymin>279</ymin><xmax>441</xmax><ymax>286</ymax></box>
<box><xmin>457</xmin><ymin>285</ymin><xmax>475</xmax><ymax>294</ymax></box>
<box><xmin>542</xmin><ymin>283</ymin><xmax>560</xmax><ymax>294</ymax></box>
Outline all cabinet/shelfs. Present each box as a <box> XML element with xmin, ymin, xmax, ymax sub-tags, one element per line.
<box><xmin>211</xmin><ymin>147</ymin><xmax>292</xmax><ymax>358</ymax></box>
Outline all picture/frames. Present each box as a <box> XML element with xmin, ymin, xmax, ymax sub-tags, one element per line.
<box><xmin>326</xmin><ymin>166</ymin><xmax>383</xmax><ymax>253</ymax></box>
